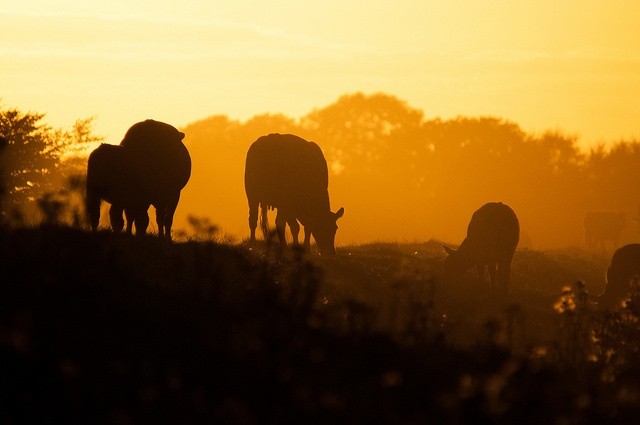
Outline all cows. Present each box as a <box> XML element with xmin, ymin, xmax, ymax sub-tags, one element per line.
<box><xmin>588</xmin><ymin>243</ymin><xmax>640</xmax><ymax>337</ymax></box>
<box><xmin>86</xmin><ymin>144</ymin><xmax>124</xmax><ymax>232</ymax></box>
<box><xmin>245</xmin><ymin>134</ymin><xmax>344</xmax><ymax>257</ymax></box>
<box><xmin>442</xmin><ymin>202</ymin><xmax>519</xmax><ymax>307</ymax></box>
<box><xmin>120</xmin><ymin>120</ymin><xmax>191</xmax><ymax>245</ymax></box>
